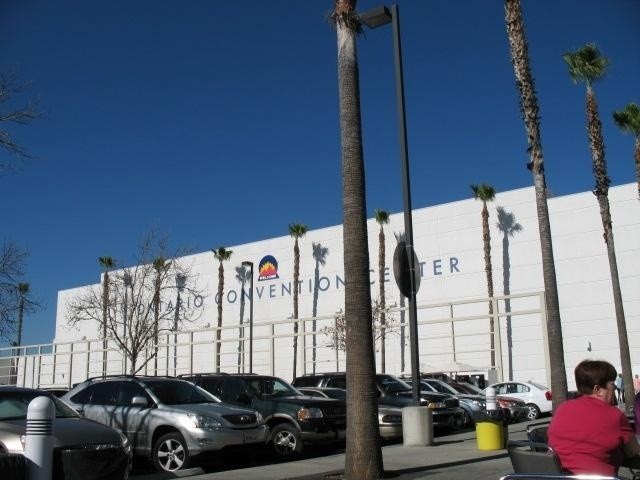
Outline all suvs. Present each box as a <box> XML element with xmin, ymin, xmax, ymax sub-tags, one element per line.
<box><xmin>291</xmin><ymin>372</ymin><xmax>467</xmax><ymax>433</ymax></box>
<box><xmin>173</xmin><ymin>372</ymin><xmax>345</xmax><ymax>458</ymax></box>
<box><xmin>56</xmin><ymin>375</ymin><xmax>271</xmax><ymax>472</ymax></box>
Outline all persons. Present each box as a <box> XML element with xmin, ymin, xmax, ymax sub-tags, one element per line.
<box><xmin>633</xmin><ymin>374</ymin><xmax>640</xmax><ymax>395</ymax></box>
<box><xmin>545</xmin><ymin>359</ymin><xmax>640</xmax><ymax>480</ymax></box>
<box><xmin>615</xmin><ymin>372</ymin><xmax>623</xmax><ymax>401</ymax></box>
<box><xmin>620</xmin><ymin>378</ymin><xmax>625</xmax><ymax>403</ymax></box>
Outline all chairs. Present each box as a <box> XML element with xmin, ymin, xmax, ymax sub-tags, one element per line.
<box><xmin>504</xmin><ymin>439</ymin><xmax>565</xmax><ymax>475</ymax></box>
<box><xmin>527</xmin><ymin>421</ymin><xmax>552</xmax><ymax>455</ymax></box>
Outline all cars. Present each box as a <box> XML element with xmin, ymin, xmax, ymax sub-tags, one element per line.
<box><xmin>1</xmin><ymin>384</ymin><xmax>134</xmax><ymax>476</ymax></box>
<box><xmin>481</xmin><ymin>380</ymin><xmax>552</xmax><ymax>421</ymax></box>
<box><xmin>273</xmin><ymin>388</ymin><xmax>403</xmax><ymax>444</ymax></box>
<box><xmin>383</xmin><ymin>378</ymin><xmax>499</xmax><ymax>430</ymax></box>
<box><xmin>448</xmin><ymin>380</ymin><xmax>528</xmax><ymax>426</ymax></box>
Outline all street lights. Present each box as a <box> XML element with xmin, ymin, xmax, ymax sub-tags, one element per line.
<box><xmin>241</xmin><ymin>261</ymin><xmax>254</xmax><ymax>375</ymax></box>
<box><xmin>358</xmin><ymin>3</ymin><xmax>435</xmax><ymax>446</ymax></box>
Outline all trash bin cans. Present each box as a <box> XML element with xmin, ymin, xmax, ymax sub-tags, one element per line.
<box><xmin>474</xmin><ymin>410</ymin><xmax>504</xmax><ymax>450</ymax></box>
